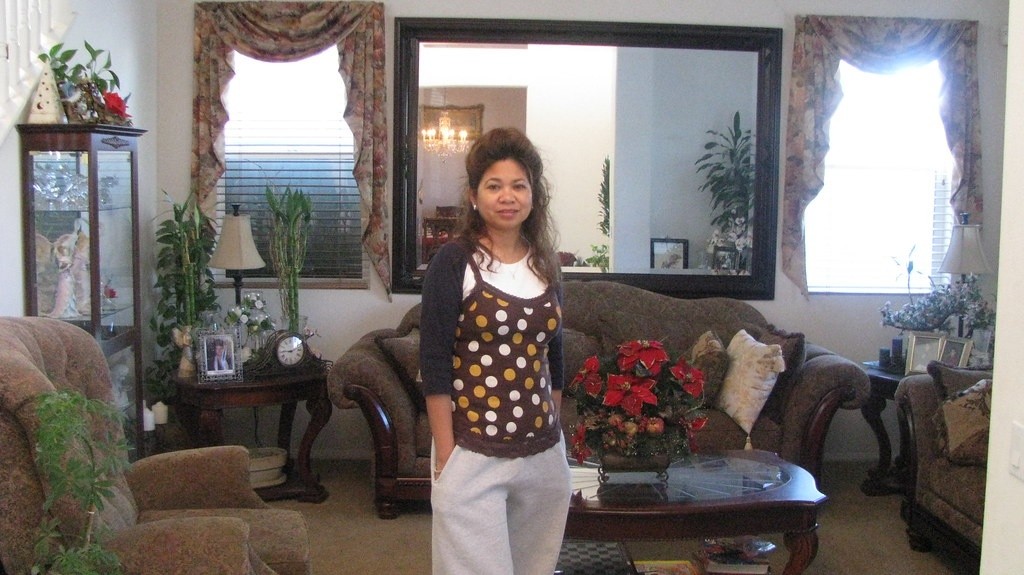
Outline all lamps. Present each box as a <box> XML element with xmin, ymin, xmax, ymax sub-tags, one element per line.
<box><xmin>938</xmin><ymin>213</ymin><xmax>992</xmax><ymax>338</ymax></box>
<box><xmin>208</xmin><ymin>204</ymin><xmax>266</xmax><ymax>304</ymax></box>
<box><xmin>422</xmin><ymin>86</ymin><xmax>469</xmax><ymax>161</ymax></box>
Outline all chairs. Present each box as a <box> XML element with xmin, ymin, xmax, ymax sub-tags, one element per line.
<box><xmin>422</xmin><ymin>205</ymin><xmax>463</xmax><ymax>263</ymax></box>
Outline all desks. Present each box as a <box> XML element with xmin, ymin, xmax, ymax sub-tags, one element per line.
<box><xmin>166</xmin><ymin>369</ymin><xmax>332</xmax><ymax>504</ymax></box>
<box><xmin>860</xmin><ymin>370</ymin><xmax>926</xmax><ymax>497</ymax></box>
<box><xmin>423</xmin><ymin>232</ymin><xmax>460</xmax><ymax>252</ymax></box>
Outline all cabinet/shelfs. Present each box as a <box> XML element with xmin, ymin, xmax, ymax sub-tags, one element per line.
<box><xmin>16</xmin><ymin>124</ymin><xmax>149</xmax><ymax>463</ymax></box>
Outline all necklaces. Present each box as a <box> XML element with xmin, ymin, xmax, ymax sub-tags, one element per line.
<box><xmin>509</xmin><ymin>262</ymin><xmax>520</xmax><ymax>279</ymax></box>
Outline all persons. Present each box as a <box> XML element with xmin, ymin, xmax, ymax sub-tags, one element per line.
<box><xmin>919</xmin><ymin>342</ymin><xmax>932</xmax><ymax>365</ymax></box>
<box><xmin>208</xmin><ymin>339</ymin><xmax>233</xmax><ymax>371</ymax></box>
<box><xmin>417</xmin><ymin>128</ymin><xmax>573</xmax><ymax>575</ymax></box>
<box><xmin>945</xmin><ymin>348</ymin><xmax>959</xmax><ymax>366</ymax></box>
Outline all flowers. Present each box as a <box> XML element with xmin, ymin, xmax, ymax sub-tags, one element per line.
<box><xmin>39</xmin><ymin>41</ymin><xmax>135</xmax><ymax>125</ymax></box>
<box><xmin>698</xmin><ymin>216</ymin><xmax>754</xmax><ymax>277</ymax></box>
<box><xmin>224</xmin><ymin>294</ymin><xmax>276</xmax><ymax>366</ymax></box>
<box><xmin>567</xmin><ymin>336</ymin><xmax>709</xmax><ymax>465</ymax></box>
<box><xmin>879</xmin><ymin>250</ymin><xmax>994</xmax><ymax>341</ymax></box>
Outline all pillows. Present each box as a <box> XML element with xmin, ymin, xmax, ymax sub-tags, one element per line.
<box><xmin>561</xmin><ymin>324</ymin><xmax>806</xmax><ymax>452</ymax></box>
<box><xmin>374</xmin><ymin>321</ymin><xmax>428</xmax><ymax>412</ymax></box>
<box><xmin>926</xmin><ymin>360</ymin><xmax>993</xmax><ymax>467</ymax></box>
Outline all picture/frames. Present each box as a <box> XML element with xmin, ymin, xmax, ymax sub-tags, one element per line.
<box><xmin>937</xmin><ymin>337</ymin><xmax>974</xmax><ymax>369</ymax></box>
<box><xmin>424</xmin><ymin>104</ymin><xmax>483</xmax><ymax>141</ymax></box>
<box><xmin>710</xmin><ymin>245</ymin><xmax>741</xmax><ymax>272</ymax></box>
<box><xmin>651</xmin><ymin>238</ymin><xmax>688</xmax><ymax>269</ymax></box>
<box><xmin>906</xmin><ymin>332</ymin><xmax>948</xmax><ymax>377</ymax></box>
<box><xmin>191</xmin><ymin>328</ymin><xmax>244</xmax><ymax>384</ymax></box>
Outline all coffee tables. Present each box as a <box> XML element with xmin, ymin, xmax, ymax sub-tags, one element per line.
<box><xmin>552</xmin><ymin>449</ymin><xmax>829</xmax><ymax>574</ymax></box>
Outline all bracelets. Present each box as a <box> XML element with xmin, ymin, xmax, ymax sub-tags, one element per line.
<box><xmin>435</xmin><ymin>469</ymin><xmax>441</xmax><ymax>474</ymax></box>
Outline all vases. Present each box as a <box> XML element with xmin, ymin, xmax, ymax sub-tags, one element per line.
<box><xmin>599</xmin><ymin>450</ymin><xmax>673</xmax><ymax>479</ymax></box>
<box><xmin>232</xmin><ymin>290</ymin><xmax>277</xmax><ymax>354</ymax></box>
<box><xmin>279</xmin><ymin>273</ymin><xmax>306</xmax><ymax>335</ymax></box>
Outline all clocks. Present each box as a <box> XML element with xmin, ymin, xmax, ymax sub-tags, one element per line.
<box><xmin>244</xmin><ymin>331</ymin><xmax>334</xmax><ymax>379</ymax></box>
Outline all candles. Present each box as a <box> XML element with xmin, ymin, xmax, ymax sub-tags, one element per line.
<box><xmin>878</xmin><ymin>338</ymin><xmax>902</xmax><ymax>369</ymax></box>
<box><xmin>143</xmin><ymin>400</ymin><xmax>169</xmax><ymax>432</ymax></box>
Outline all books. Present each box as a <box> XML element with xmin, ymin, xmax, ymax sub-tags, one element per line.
<box><xmin>702</xmin><ymin>557</ymin><xmax>770</xmax><ymax>575</ymax></box>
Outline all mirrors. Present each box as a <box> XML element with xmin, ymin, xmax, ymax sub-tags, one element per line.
<box><xmin>391</xmin><ymin>16</ymin><xmax>783</xmax><ymax>302</ymax></box>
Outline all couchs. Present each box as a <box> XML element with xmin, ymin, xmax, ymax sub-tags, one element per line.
<box><xmin>894</xmin><ymin>360</ymin><xmax>995</xmax><ymax>569</ymax></box>
<box><xmin>0</xmin><ymin>317</ymin><xmax>312</xmax><ymax>575</ymax></box>
<box><xmin>328</xmin><ymin>281</ymin><xmax>870</xmax><ymax>521</ymax></box>
